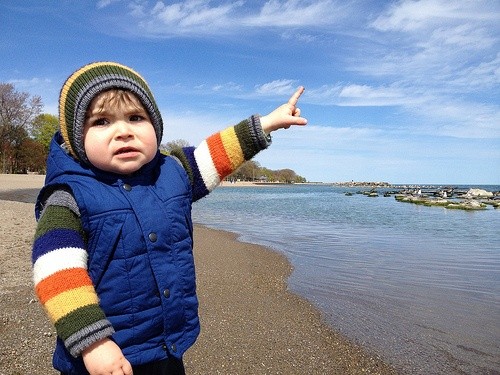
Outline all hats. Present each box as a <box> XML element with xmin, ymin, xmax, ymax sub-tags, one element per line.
<box><xmin>58</xmin><ymin>60</ymin><xmax>164</xmax><ymax>169</ymax></box>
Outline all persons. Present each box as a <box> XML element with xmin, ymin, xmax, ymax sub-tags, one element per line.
<box><xmin>32</xmin><ymin>60</ymin><xmax>308</xmax><ymax>375</ymax></box>
